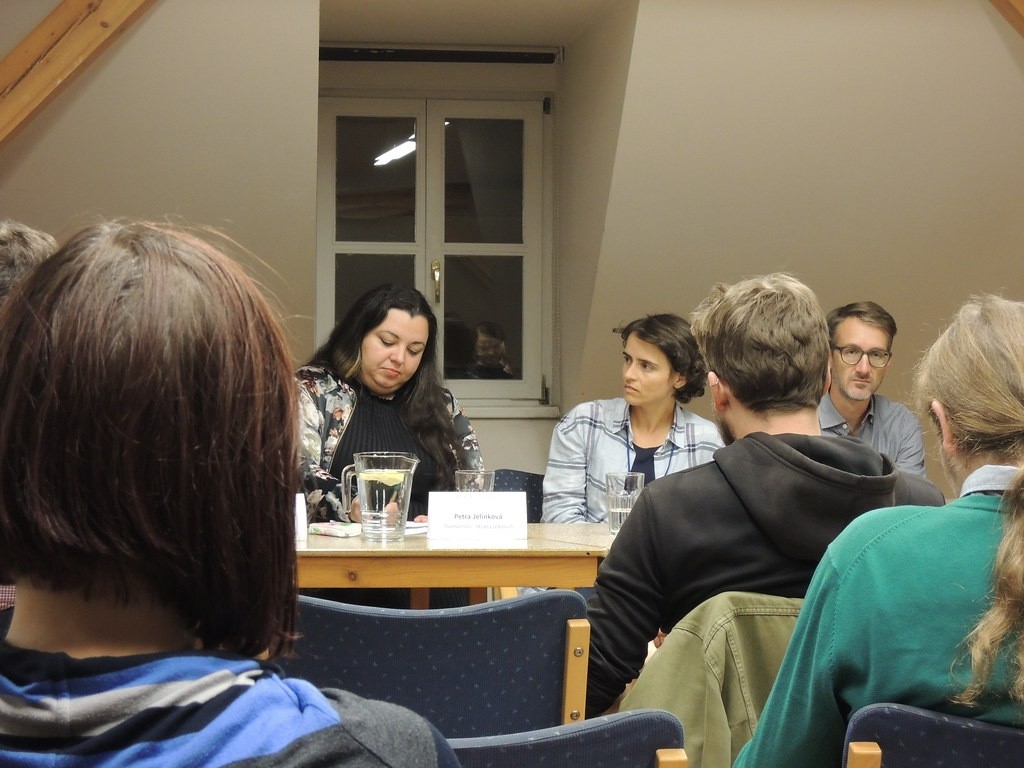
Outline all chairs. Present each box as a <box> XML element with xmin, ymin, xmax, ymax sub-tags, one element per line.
<box><xmin>492</xmin><ymin>469</ymin><xmax>545</xmax><ymax>523</ymax></box>
<box><xmin>272</xmin><ymin>590</ymin><xmax>1023</xmax><ymax>768</ymax></box>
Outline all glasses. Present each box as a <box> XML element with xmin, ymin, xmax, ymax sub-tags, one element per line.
<box><xmin>832</xmin><ymin>344</ymin><xmax>895</xmax><ymax>368</ymax></box>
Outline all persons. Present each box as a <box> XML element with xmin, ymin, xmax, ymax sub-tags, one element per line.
<box><xmin>292</xmin><ymin>286</ymin><xmax>482</xmax><ymax>610</ymax></box>
<box><xmin>584</xmin><ymin>273</ymin><xmax>944</xmax><ymax>723</ymax></box>
<box><xmin>522</xmin><ymin>313</ymin><xmax>727</xmax><ymax>595</ymax></box>
<box><xmin>732</xmin><ymin>296</ymin><xmax>1024</xmax><ymax>768</ymax></box>
<box><xmin>818</xmin><ymin>303</ymin><xmax>927</xmax><ymax>482</ymax></box>
<box><xmin>1</xmin><ymin>219</ymin><xmax>461</xmax><ymax>768</ymax></box>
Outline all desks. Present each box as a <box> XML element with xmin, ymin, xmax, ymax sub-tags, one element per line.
<box><xmin>294</xmin><ymin>523</ymin><xmax>619</xmax><ymax>610</ymax></box>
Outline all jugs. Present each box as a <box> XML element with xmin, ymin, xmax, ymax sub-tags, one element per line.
<box><xmin>340</xmin><ymin>451</ymin><xmax>421</xmax><ymax>544</ymax></box>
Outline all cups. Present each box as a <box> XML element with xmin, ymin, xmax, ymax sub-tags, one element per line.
<box><xmin>605</xmin><ymin>471</ymin><xmax>646</xmax><ymax>535</ymax></box>
<box><xmin>454</xmin><ymin>471</ymin><xmax>497</xmax><ymax>492</ymax></box>
